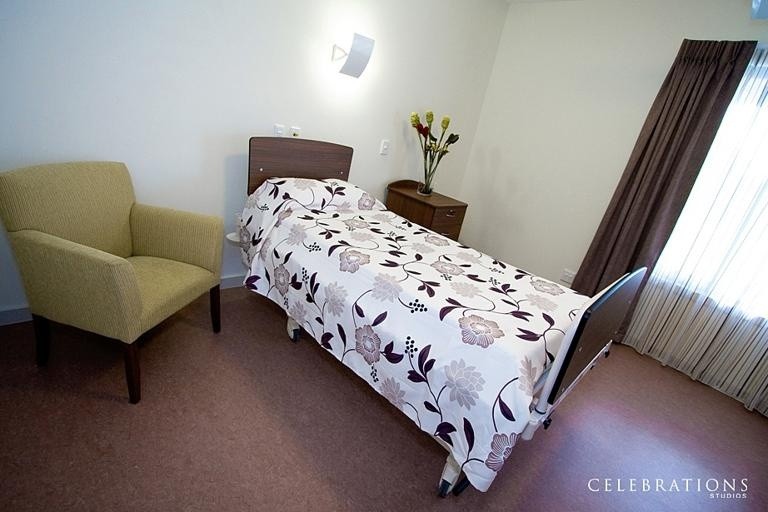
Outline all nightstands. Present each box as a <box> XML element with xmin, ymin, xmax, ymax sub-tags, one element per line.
<box><xmin>385</xmin><ymin>180</ymin><xmax>468</xmax><ymax>242</ymax></box>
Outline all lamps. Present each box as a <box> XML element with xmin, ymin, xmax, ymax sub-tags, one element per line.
<box><xmin>330</xmin><ymin>32</ymin><xmax>375</xmax><ymax>79</ymax></box>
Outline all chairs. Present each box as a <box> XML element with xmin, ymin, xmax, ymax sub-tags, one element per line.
<box><xmin>0</xmin><ymin>161</ymin><xmax>224</xmax><ymax>405</ymax></box>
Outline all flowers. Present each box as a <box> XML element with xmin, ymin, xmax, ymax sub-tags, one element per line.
<box><xmin>409</xmin><ymin>110</ymin><xmax>460</xmax><ymax>194</ymax></box>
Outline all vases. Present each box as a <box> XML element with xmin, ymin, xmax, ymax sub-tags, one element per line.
<box><xmin>417</xmin><ymin>159</ymin><xmax>443</xmax><ymax>197</ymax></box>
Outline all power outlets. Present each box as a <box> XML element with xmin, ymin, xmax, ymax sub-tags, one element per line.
<box><xmin>380</xmin><ymin>140</ymin><xmax>392</xmax><ymax>155</ymax></box>
<box><xmin>560</xmin><ymin>268</ymin><xmax>576</xmax><ymax>284</ymax></box>
<box><xmin>239</xmin><ymin>136</ymin><xmax>650</xmax><ymax>501</ymax></box>
